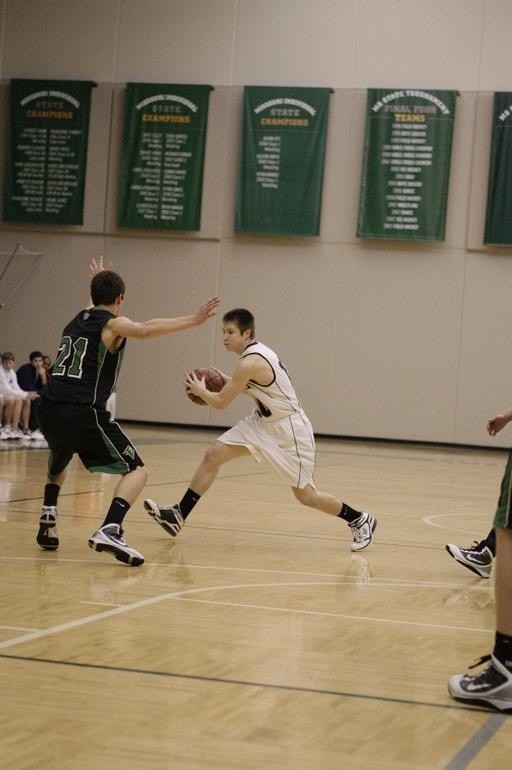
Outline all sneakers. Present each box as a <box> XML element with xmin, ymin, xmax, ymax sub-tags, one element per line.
<box><xmin>0</xmin><ymin>425</ymin><xmax>45</xmax><ymax>440</ymax></box>
<box><xmin>37</xmin><ymin>506</ymin><xmax>58</xmax><ymax>551</ymax></box>
<box><xmin>448</xmin><ymin>653</ymin><xmax>511</xmax><ymax>712</ymax></box>
<box><xmin>89</xmin><ymin>522</ymin><xmax>144</xmax><ymax>565</ymax></box>
<box><xmin>144</xmin><ymin>500</ymin><xmax>184</xmax><ymax>537</ymax></box>
<box><xmin>349</xmin><ymin>513</ymin><xmax>377</xmax><ymax>551</ymax></box>
<box><xmin>446</xmin><ymin>541</ymin><xmax>494</xmax><ymax>579</ymax></box>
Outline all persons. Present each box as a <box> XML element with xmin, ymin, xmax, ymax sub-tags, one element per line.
<box><xmin>445</xmin><ymin>407</ymin><xmax>512</xmax><ymax>580</ymax></box>
<box><xmin>36</xmin><ymin>256</ymin><xmax>219</xmax><ymax>567</ymax></box>
<box><xmin>445</xmin><ymin>450</ymin><xmax>511</xmax><ymax>714</ymax></box>
<box><xmin>0</xmin><ymin>351</ymin><xmax>54</xmax><ymax>440</ymax></box>
<box><xmin>143</xmin><ymin>306</ymin><xmax>378</xmax><ymax>553</ymax></box>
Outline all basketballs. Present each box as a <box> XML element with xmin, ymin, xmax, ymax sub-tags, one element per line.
<box><xmin>189</xmin><ymin>369</ymin><xmax>223</xmax><ymax>404</ymax></box>
